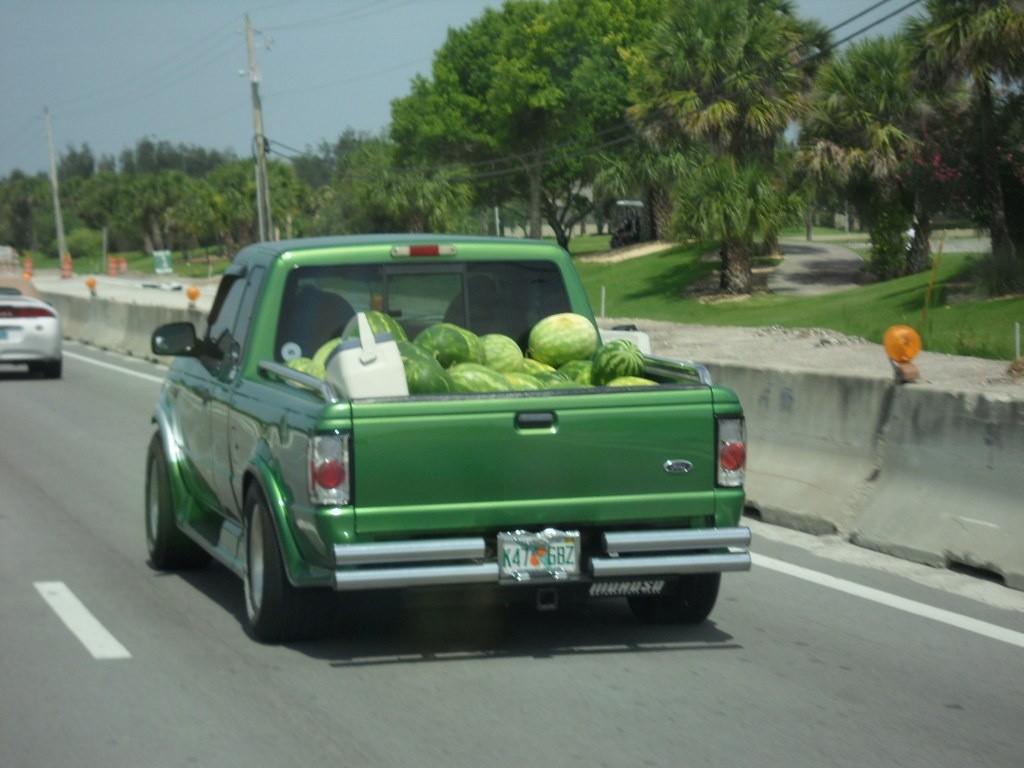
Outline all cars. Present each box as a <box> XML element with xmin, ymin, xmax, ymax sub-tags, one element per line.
<box><xmin>0</xmin><ymin>286</ymin><xmax>62</xmax><ymax>380</ymax></box>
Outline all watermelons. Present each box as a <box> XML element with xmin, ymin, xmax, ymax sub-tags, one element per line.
<box><xmin>282</xmin><ymin>310</ymin><xmax>660</xmax><ymax>396</ymax></box>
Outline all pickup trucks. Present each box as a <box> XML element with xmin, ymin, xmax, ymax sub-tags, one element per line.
<box><xmin>145</xmin><ymin>233</ymin><xmax>752</xmax><ymax>643</ymax></box>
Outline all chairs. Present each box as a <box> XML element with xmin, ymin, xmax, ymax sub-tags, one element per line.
<box><xmin>442</xmin><ymin>289</ymin><xmax>548</xmax><ymax>359</ymax></box>
<box><xmin>273</xmin><ymin>289</ymin><xmax>356</xmax><ymax>366</ymax></box>
